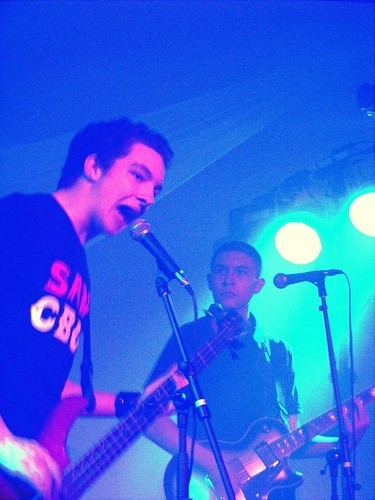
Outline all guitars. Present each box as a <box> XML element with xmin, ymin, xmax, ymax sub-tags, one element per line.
<box><xmin>163</xmin><ymin>385</ymin><xmax>375</xmax><ymax>500</ymax></box>
<box><xmin>0</xmin><ymin>304</ymin><xmax>248</xmax><ymax>500</ymax></box>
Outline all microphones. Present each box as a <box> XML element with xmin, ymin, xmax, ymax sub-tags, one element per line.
<box><xmin>128</xmin><ymin>218</ymin><xmax>194</xmax><ymax>296</ymax></box>
<box><xmin>273</xmin><ymin>269</ymin><xmax>345</xmax><ymax>289</ymax></box>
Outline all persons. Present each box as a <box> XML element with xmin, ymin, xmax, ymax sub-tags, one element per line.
<box><xmin>139</xmin><ymin>241</ymin><xmax>369</xmax><ymax>500</ymax></box>
<box><xmin>0</xmin><ymin>116</ymin><xmax>189</xmax><ymax>500</ymax></box>
